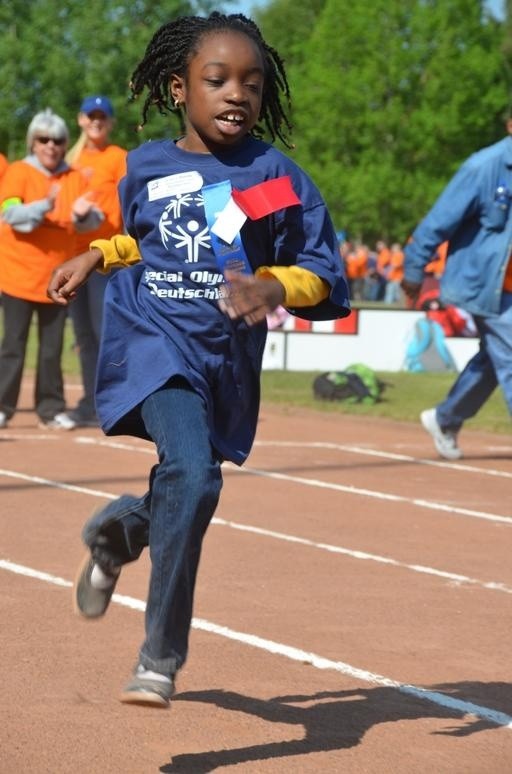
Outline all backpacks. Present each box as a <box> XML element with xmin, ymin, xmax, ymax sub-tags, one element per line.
<box><xmin>312</xmin><ymin>363</ymin><xmax>394</xmax><ymax>404</ymax></box>
<box><xmin>400</xmin><ymin>318</ymin><xmax>456</xmax><ymax>372</ymax></box>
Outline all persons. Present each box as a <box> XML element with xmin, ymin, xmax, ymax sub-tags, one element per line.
<box><xmin>63</xmin><ymin>96</ymin><xmax>128</xmax><ymax>427</ymax></box>
<box><xmin>47</xmin><ymin>10</ymin><xmax>352</xmax><ymax>708</ymax></box>
<box><xmin>401</xmin><ymin>117</ymin><xmax>512</xmax><ymax>461</ymax></box>
<box><xmin>338</xmin><ymin>234</ymin><xmax>448</xmax><ymax>303</ymax></box>
<box><xmin>0</xmin><ymin>108</ymin><xmax>105</xmax><ymax>430</ymax></box>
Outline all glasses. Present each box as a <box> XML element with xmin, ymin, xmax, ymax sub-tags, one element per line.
<box><xmin>34</xmin><ymin>136</ymin><xmax>65</xmax><ymax>145</ymax></box>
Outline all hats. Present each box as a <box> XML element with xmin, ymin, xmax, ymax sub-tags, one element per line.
<box><xmin>82</xmin><ymin>96</ymin><xmax>112</xmax><ymax>116</ymax></box>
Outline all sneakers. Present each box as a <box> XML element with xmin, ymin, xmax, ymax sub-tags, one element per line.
<box><xmin>37</xmin><ymin>409</ymin><xmax>100</xmax><ymax>432</ymax></box>
<box><xmin>119</xmin><ymin>658</ymin><xmax>174</xmax><ymax>709</ymax></box>
<box><xmin>0</xmin><ymin>410</ymin><xmax>7</xmax><ymax>428</ymax></box>
<box><xmin>72</xmin><ymin>547</ymin><xmax>121</xmax><ymax>617</ymax></box>
<box><xmin>422</xmin><ymin>410</ymin><xmax>463</xmax><ymax>460</ymax></box>
